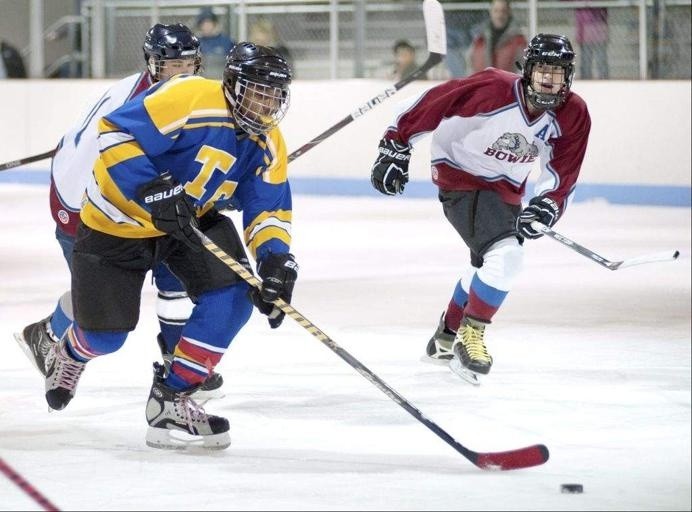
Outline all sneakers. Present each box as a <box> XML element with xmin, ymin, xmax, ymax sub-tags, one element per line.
<box><xmin>425</xmin><ymin>314</ymin><xmax>458</xmax><ymax>359</ymax></box>
<box><xmin>25</xmin><ymin>315</ymin><xmax>58</xmax><ymax>373</ymax></box>
<box><xmin>143</xmin><ymin>360</ymin><xmax>231</xmax><ymax>435</ymax></box>
<box><xmin>456</xmin><ymin>310</ymin><xmax>496</xmax><ymax>374</ymax></box>
<box><xmin>156</xmin><ymin>328</ymin><xmax>227</xmax><ymax>390</ymax></box>
<box><xmin>41</xmin><ymin>335</ymin><xmax>90</xmax><ymax>412</ymax></box>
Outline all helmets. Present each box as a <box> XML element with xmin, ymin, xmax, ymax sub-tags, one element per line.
<box><xmin>142</xmin><ymin>22</ymin><xmax>200</xmax><ymax>80</ymax></box>
<box><xmin>521</xmin><ymin>32</ymin><xmax>575</xmax><ymax>110</ymax></box>
<box><xmin>220</xmin><ymin>43</ymin><xmax>291</xmax><ymax>137</ymax></box>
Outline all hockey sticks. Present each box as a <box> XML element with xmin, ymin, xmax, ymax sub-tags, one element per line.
<box><xmin>530</xmin><ymin>217</ymin><xmax>679</xmax><ymax>272</ymax></box>
<box><xmin>286</xmin><ymin>0</ymin><xmax>446</xmax><ymax>165</ymax></box>
<box><xmin>190</xmin><ymin>225</ymin><xmax>549</xmax><ymax>472</ymax></box>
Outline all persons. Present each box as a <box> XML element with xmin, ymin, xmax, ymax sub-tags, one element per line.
<box><xmin>46</xmin><ymin>46</ymin><xmax>83</xmax><ymax>80</ymax></box>
<box><xmin>0</xmin><ymin>43</ymin><xmax>27</xmax><ymax>79</ymax></box>
<box><xmin>471</xmin><ymin>0</ymin><xmax>527</xmax><ymax>75</ymax></box>
<box><xmin>246</xmin><ymin>20</ymin><xmax>292</xmax><ymax>63</ymax></box>
<box><xmin>389</xmin><ymin>40</ymin><xmax>428</xmax><ymax>81</ymax></box>
<box><xmin>371</xmin><ymin>33</ymin><xmax>593</xmax><ymax>388</ymax></box>
<box><xmin>10</xmin><ymin>21</ymin><xmax>224</xmax><ymax>401</ymax></box>
<box><xmin>42</xmin><ymin>41</ymin><xmax>300</xmax><ymax>458</ymax></box>
<box><xmin>195</xmin><ymin>9</ymin><xmax>232</xmax><ymax>78</ymax></box>
<box><xmin>575</xmin><ymin>0</ymin><xmax>677</xmax><ymax>81</ymax></box>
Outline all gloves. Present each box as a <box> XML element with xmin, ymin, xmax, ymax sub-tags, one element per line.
<box><xmin>253</xmin><ymin>251</ymin><xmax>300</xmax><ymax>326</ymax></box>
<box><xmin>514</xmin><ymin>193</ymin><xmax>558</xmax><ymax>243</ymax></box>
<box><xmin>369</xmin><ymin>134</ymin><xmax>410</xmax><ymax>197</ymax></box>
<box><xmin>139</xmin><ymin>175</ymin><xmax>200</xmax><ymax>244</ymax></box>
